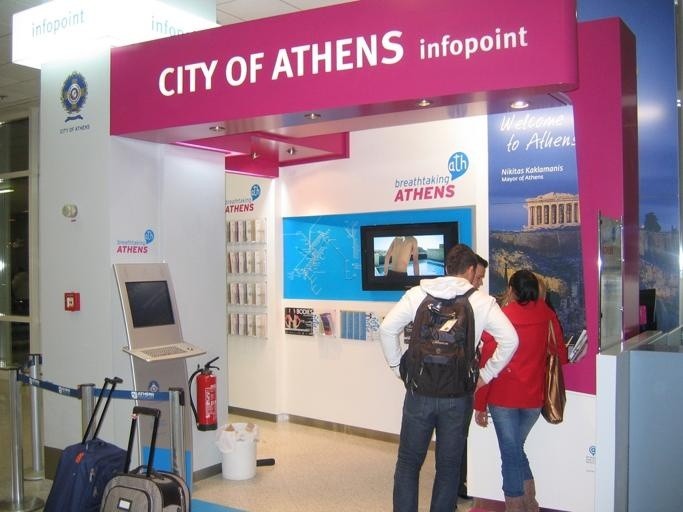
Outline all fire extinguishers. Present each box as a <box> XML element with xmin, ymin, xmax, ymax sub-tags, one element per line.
<box><xmin>188</xmin><ymin>356</ymin><xmax>220</xmax><ymax>432</ymax></box>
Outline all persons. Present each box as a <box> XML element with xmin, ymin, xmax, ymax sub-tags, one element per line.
<box><xmin>383</xmin><ymin>235</ymin><xmax>420</xmax><ymax>277</ymax></box>
<box><xmin>284</xmin><ymin>308</ymin><xmax>301</xmax><ymax>329</ymax></box>
<box><xmin>457</xmin><ymin>254</ymin><xmax>489</xmax><ymax>502</ymax></box>
<box><xmin>377</xmin><ymin>242</ymin><xmax>519</xmax><ymax>512</ymax></box>
<box><xmin>469</xmin><ymin>269</ymin><xmax>569</xmax><ymax>512</ymax></box>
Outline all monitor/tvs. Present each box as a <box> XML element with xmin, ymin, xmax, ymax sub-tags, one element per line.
<box><xmin>125</xmin><ymin>280</ymin><xmax>176</xmax><ymax>328</ymax></box>
<box><xmin>359</xmin><ymin>221</ymin><xmax>459</xmax><ymax>291</ymax></box>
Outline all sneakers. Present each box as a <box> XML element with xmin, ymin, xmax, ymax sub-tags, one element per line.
<box><xmin>457</xmin><ymin>480</ymin><xmax>473</xmax><ymax>500</ymax></box>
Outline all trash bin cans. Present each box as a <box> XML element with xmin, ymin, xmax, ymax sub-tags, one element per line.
<box><xmin>217</xmin><ymin>423</ymin><xmax>258</xmax><ymax>480</ymax></box>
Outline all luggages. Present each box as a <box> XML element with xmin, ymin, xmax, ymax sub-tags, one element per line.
<box><xmin>40</xmin><ymin>373</ymin><xmax>129</xmax><ymax>512</ymax></box>
<box><xmin>97</xmin><ymin>403</ymin><xmax>191</xmax><ymax>512</ymax></box>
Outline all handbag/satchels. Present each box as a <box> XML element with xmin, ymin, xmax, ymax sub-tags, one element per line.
<box><xmin>539</xmin><ymin>350</ymin><xmax>568</xmax><ymax>426</ymax></box>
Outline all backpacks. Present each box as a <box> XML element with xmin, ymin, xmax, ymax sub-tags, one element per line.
<box><xmin>395</xmin><ymin>286</ymin><xmax>483</xmax><ymax>400</ymax></box>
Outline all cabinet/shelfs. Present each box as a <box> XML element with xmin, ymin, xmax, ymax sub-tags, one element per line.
<box><xmin>227</xmin><ymin>242</ymin><xmax>267</xmax><ymax>341</ymax></box>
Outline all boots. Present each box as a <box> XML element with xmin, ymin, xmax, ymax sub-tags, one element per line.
<box><xmin>502</xmin><ymin>492</ymin><xmax>532</xmax><ymax>512</ymax></box>
<box><xmin>521</xmin><ymin>479</ymin><xmax>539</xmax><ymax>512</ymax></box>
